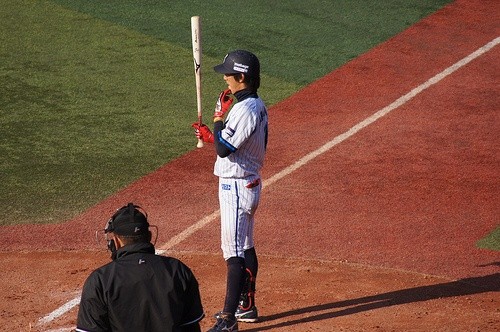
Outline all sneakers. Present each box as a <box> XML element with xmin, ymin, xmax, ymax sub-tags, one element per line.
<box><xmin>214</xmin><ymin>305</ymin><xmax>258</xmax><ymax>323</ymax></box>
<box><xmin>206</xmin><ymin>312</ymin><xmax>238</xmax><ymax>332</ymax></box>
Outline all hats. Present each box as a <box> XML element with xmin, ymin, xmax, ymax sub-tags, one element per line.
<box><xmin>105</xmin><ymin>203</ymin><xmax>149</xmax><ymax>235</ymax></box>
<box><xmin>214</xmin><ymin>50</ymin><xmax>260</xmax><ymax>75</ymax></box>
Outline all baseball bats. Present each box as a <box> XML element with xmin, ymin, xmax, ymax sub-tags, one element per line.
<box><xmin>190</xmin><ymin>16</ymin><xmax>205</xmax><ymax>148</ymax></box>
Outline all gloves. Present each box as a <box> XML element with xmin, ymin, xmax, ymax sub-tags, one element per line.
<box><xmin>213</xmin><ymin>89</ymin><xmax>233</xmax><ymax>118</ymax></box>
<box><xmin>192</xmin><ymin>123</ymin><xmax>214</xmax><ymax>144</ymax></box>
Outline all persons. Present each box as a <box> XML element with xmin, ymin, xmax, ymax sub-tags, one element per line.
<box><xmin>192</xmin><ymin>51</ymin><xmax>268</xmax><ymax>330</ymax></box>
<box><xmin>74</xmin><ymin>202</ymin><xmax>205</xmax><ymax>332</ymax></box>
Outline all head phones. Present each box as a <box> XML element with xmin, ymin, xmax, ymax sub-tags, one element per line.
<box><xmin>104</xmin><ymin>205</ymin><xmax>152</xmax><ymax>249</ymax></box>
